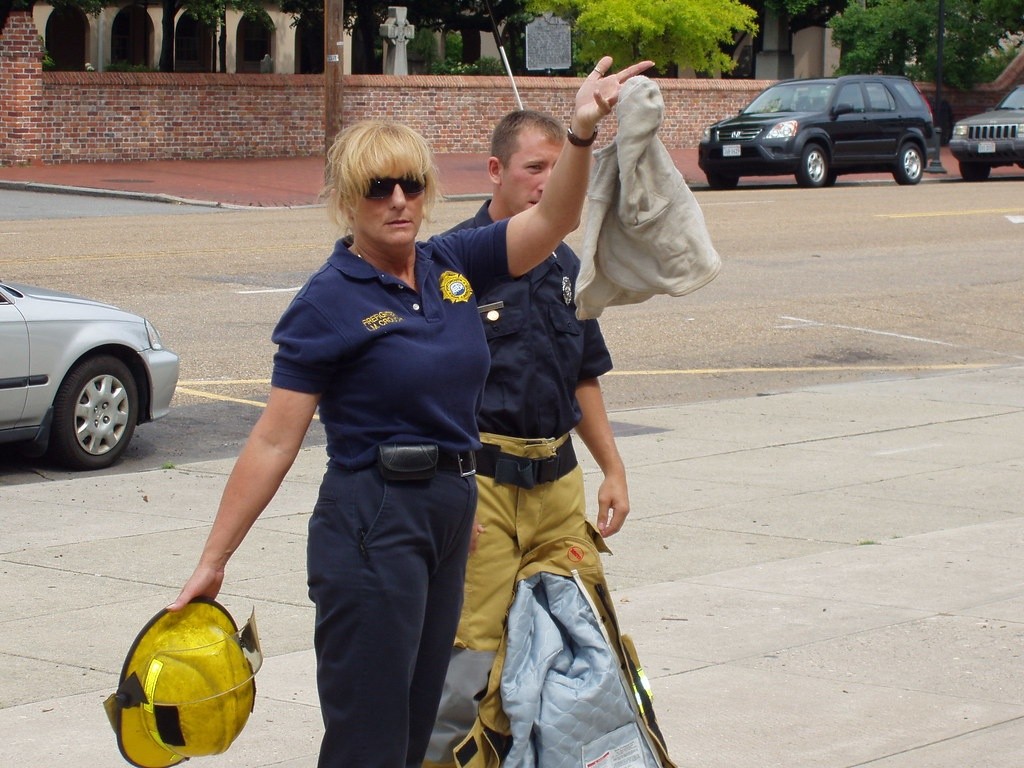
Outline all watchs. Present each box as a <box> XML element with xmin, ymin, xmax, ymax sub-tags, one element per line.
<box><xmin>567</xmin><ymin>125</ymin><xmax>598</xmax><ymax>146</ymax></box>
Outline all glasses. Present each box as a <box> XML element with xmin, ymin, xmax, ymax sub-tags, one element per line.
<box><xmin>365</xmin><ymin>176</ymin><xmax>424</xmax><ymax>199</ymax></box>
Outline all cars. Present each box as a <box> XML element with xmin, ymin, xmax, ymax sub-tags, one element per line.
<box><xmin>950</xmin><ymin>86</ymin><xmax>1024</xmax><ymax>180</ymax></box>
<box><xmin>0</xmin><ymin>282</ymin><xmax>183</xmax><ymax>474</ymax></box>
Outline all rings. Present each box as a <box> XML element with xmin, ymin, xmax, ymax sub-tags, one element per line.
<box><xmin>594</xmin><ymin>67</ymin><xmax>605</xmax><ymax>76</ymax></box>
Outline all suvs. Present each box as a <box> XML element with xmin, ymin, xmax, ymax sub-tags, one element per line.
<box><xmin>698</xmin><ymin>74</ymin><xmax>935</xmax><ymax>189</ymax></box>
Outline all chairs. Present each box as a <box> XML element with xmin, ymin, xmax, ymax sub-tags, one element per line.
<box><xmin>796</xmin><ymin>95</ymin><xmax>824</xmax><ymax>110</ymax></box>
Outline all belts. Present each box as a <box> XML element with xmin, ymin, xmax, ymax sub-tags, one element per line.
<box><xmin>437</xmin><ymin>447</ymin><xmax>477</xmax><ymax>477</ymax></box>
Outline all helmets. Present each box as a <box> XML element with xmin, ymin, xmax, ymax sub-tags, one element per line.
<box><xmin>103</xmin><ymin>597</ymin><xmax>263</xmax><ymax>768</ymax></box>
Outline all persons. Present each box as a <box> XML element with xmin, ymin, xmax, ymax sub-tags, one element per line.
<box><xmin>166</xmin><ymin>55</ymin><xmax>677</xmax><ymax>768</ymax></box>
<box><xmin>420</xmin><ymin>110</ymin><xmax>631</xmax><ymax>768</ymax></box>
